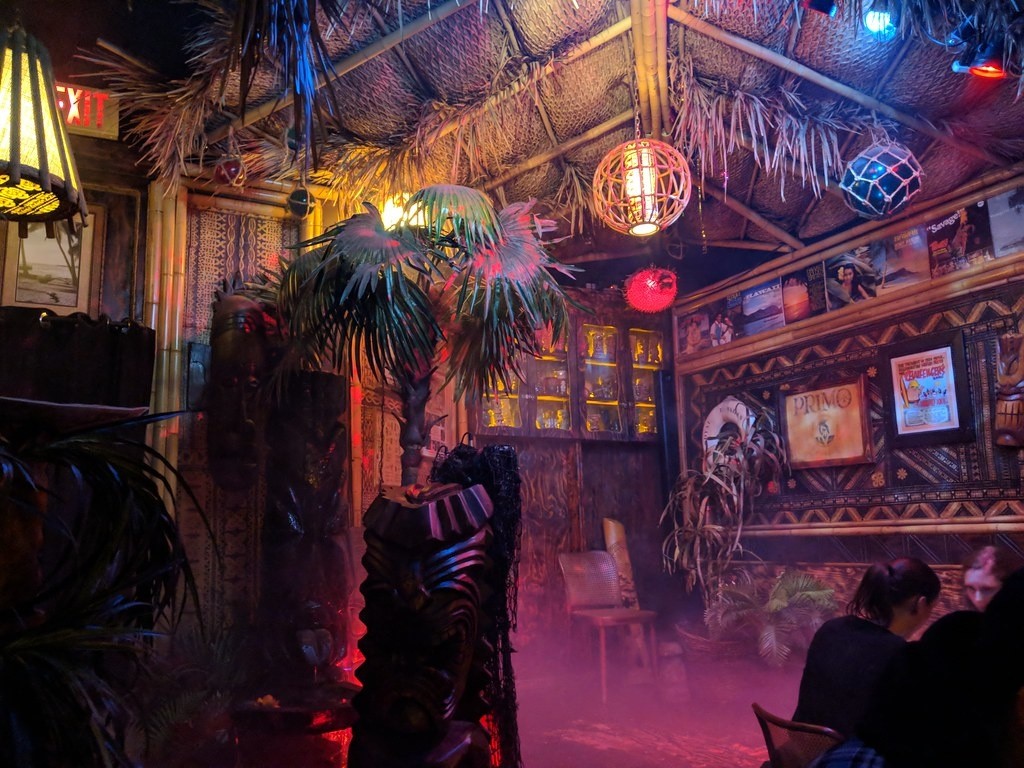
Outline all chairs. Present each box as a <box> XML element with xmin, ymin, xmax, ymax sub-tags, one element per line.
<box><xmin>752</xmin><ymin>702</ymin><xmax>843</xmax><ymax>768</ymax></box>
<box><xmin>558</xmin><ymin>549</ymin><xmax>660</xmax><ymax>709</ymax></box>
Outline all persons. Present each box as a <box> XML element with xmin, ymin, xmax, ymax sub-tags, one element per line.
<box><xmin>681</xmin><ymin>316</ymin><xmax>709</xmax><ymax>353</ymax></box>
<box><xmin>922</xmin><ymin>544</ymin><xmax>1024</xmax><ymax>768</ymax></box>
<box><xmin>826</xmin><ymin>260</ymin><xmax>873</xmax><ymax>310</ymax></box>
<box><xmin>711</xmin><ymin>311</ymin><xmax>734</xmax><ymax>346</ymax></box>
<box><xmin>792</xmin><ymin>556</ymin><xmax>941</xmax><ymax>722</ymax></box>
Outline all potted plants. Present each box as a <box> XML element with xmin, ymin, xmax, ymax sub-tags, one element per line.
<box><xmin>653</xmin><ymin>408</ymin><xmax>835</xmax><ymax>708</ymax></box>
<box><xmin>230</xmin><ymin>372</ymin><xmax>361</xmax><ymax>768</ymax></box>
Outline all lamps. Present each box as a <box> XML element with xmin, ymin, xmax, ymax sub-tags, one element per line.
<box><xmin>592</xmin><ymin>89</ymin><xmax>691</xmax><ymax>236</ymax></box>
<box><xmin>624</xmin><ymin>262</ymin><xmax>679</xmax><ymax>314</ymax></box>
<box><xmin>0</xmin><ymin>0</ymin><xmax>89</xmax><ymax>239</ymax></box>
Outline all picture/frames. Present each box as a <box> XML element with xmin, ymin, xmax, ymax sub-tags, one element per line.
<box><xmin>777</xmin><ymin>372</ymin><xmax>874</xmax><ymax>469</ymax></box>
<box><xmin>879</xmin><ymin>331</ymin><xmax>977</xmax><ymax>448</ymax></box>
<box><xmin>1</xmin><ymin>201</ymin><xmax>107</xmax><ymax>322</ymax></box>
